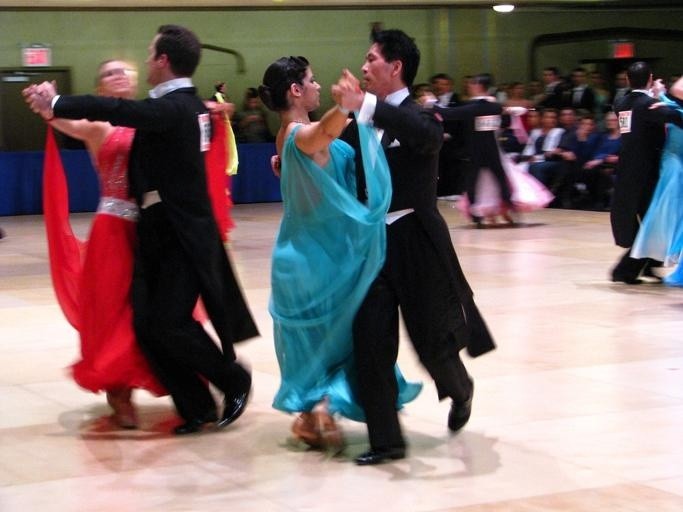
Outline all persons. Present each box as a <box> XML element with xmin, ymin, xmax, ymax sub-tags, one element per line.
<box><xmin>23</xmin><ymin>58</ymin><xmax>236</xmax><ymax>432</ymax></box>
<box><xmin>209</xmin><ymin>66</ymin><xmax>681</xmax><ymax>229</ymax></box>
<box><xmin>33</xmin><ymin>23</ymin><xmax>260</xmax><ymax>437</ymax></box>
<box><xmin>255</xmin><ymin>55</ymin><xmax>363</xmax><ymax>453</ymax></box>
<box><xmin>268</xmin><ymin>23</ymin><xmax>497</xmax><ymax>467</ymax></box>
<box><xmin>607</xmin><ymin>61</ymin><xmax>681</xmax><ymax>287</ymax></box>
<box><xmin>628</xmin><ymin>73</ymin><xmax>682</xmax><ymax>286</ymax></box>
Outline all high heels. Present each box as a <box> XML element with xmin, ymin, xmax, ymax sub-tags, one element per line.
<box><xmin>292</xmin><ymin>403</ymin><xmax>347</xmax><ymax>448</ymax></box>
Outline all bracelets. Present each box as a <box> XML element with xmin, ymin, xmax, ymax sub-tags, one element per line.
<box><xmin>338</xmin><ymin>104</ymin><xmax>349</xmax><ymax>115</ymax></box>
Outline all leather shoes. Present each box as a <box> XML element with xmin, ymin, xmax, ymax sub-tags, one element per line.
<box><xmin>108</xmin><ymin>395</ymin><xmax>138</xmax><ymax>427</ymax></box>
<box><xmin>613</xmin><ymin>270</ymin><xmax>661</xmax><ymax>284</ymax></box>
<box><xmin>449</xmin><ymin>376</ymin><xmax>474</xmax><ymax>431</ymax></box>
<box><xmin>176</xmin><ymin>406</ymin><xmax>218</xmax><ymax>436</ymax></box>
<box><xmin>218</xmin><ymin>372</ymin><xmax>251</xmax><ymax>428</ymax></box>
<box><xmin>355</xmin><ymin>442</ymin><xmax>405</xmax><ymax>464</ymax></box>
